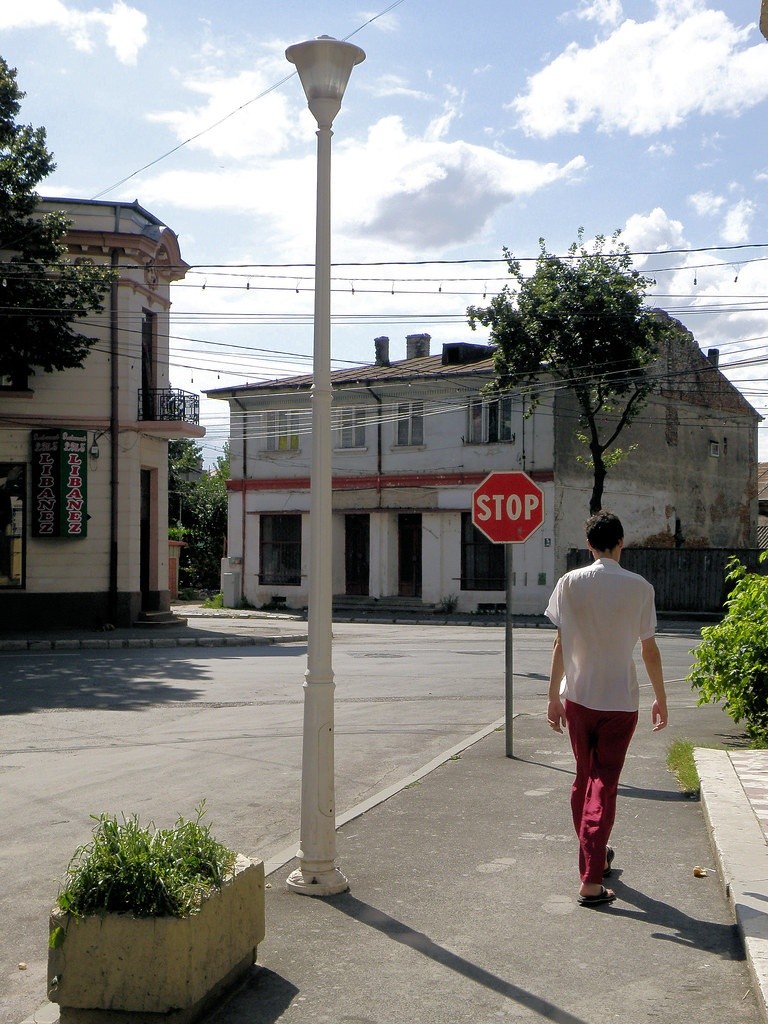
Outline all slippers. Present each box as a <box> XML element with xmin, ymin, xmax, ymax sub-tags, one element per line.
<box><xmin>599</xmin><ymin>844</ymin><xmax>615</xmax><ymax>878</ymax></box>
<box><xmin>577</xmin><ymin>884</ymin><xmax>616</xmax><ymax>904</ymax></box>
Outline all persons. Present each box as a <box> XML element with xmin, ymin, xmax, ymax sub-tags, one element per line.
<box><xmin>545</xmin><ymin>511</ymin><xmax>669</xmax><ymax>902</ymax></box>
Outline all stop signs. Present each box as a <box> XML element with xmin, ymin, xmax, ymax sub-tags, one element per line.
<box><xmin>471</xmin><ymin>469</ymin><xmax>543</xmax><ymax>544</ymax></box>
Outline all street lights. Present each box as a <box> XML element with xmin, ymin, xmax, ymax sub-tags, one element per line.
<box><xmin>286</xmin><ymin>27</ymin><xmax>370</xmax><ymax>900</ymax></box>
